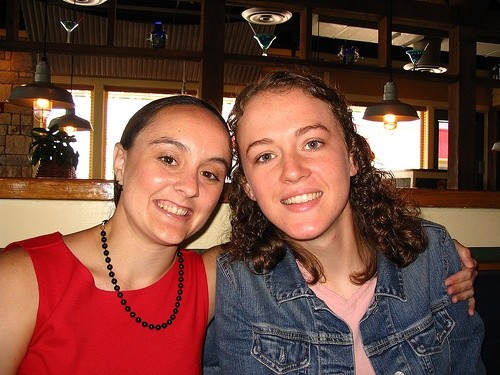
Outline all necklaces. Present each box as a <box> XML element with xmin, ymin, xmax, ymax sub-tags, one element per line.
<box><xmin>100</xmin><ymin>220</ymin><xmax>186</xmax><ymax>330</ymax></box>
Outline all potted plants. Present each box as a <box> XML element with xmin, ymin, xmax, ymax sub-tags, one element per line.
<box><xmin>29</xmin><ymin>125</ymin><xmax>79</xmax><ymax>178</ymax></box>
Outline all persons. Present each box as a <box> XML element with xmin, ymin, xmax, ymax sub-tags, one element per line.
<box><xmin>202</xmin><ymin>71</ymin><xmax>486</xmax><ymax>375</ymax></box>
<box><xmin>0</xmin><ymin>95</ymin><xmax>478</xmax><ymax>375</ymax></box>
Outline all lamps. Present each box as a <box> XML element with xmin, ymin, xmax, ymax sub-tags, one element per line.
<box><xmin>6</xmin><ymin>0</ymin><xmax>75</xmax><ymax>124</ymax></box>
<box><xmin>363</xmin><ymin>0</ymin><xmax>420</xmax><ymax>132</ymax></box>
<box><xmin>59</xmin><ymin>0</ymin><xmax>107</xmax><ymax>46</ymax></box>
<box><xmin>48</xmin><ymin>0</ymin><xmax>94</xmax><ymax>138</ymax></box>
<box><xmin>337</xmin><ymin>43</ymin><xmax>360</xmax><ymax>66</ymax></box>
<box><xmin>147</xmin><ymin>21</ymin><xmax>168</xmax><ymax>52</ymax></box>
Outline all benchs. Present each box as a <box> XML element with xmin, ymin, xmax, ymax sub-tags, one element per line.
<box><xmin>1</xmin><ymin>177</ymin><xmax>500</xmax><ymax>374</ymax></box>
